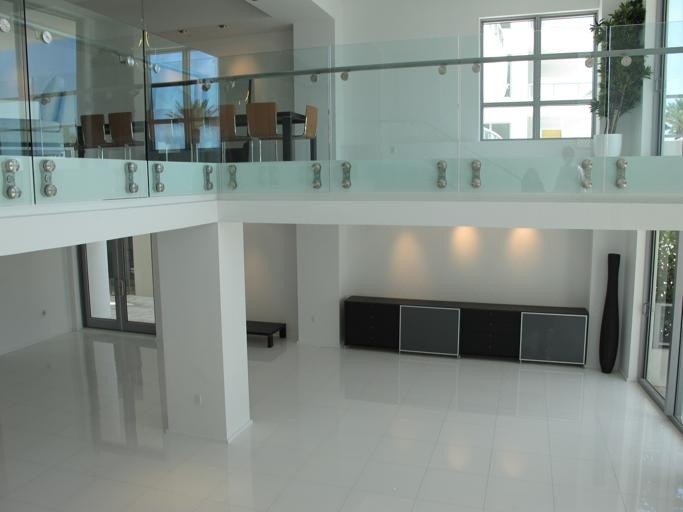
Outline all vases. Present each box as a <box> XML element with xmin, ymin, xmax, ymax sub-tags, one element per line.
<box><xmin>599</xmin><ymin>252</ymin><xmax>622</xmax><ymax>372</ymax></box>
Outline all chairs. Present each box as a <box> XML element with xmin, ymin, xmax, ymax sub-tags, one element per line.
<box><xmin>70</xmin><ymin>103</ymin><xmax>317</xmax><ymax>164</ymax></box>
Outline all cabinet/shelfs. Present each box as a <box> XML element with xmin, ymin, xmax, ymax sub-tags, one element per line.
<box><xmin>343</xmin><ymin>293</ymin><xmax>589</xmax><ymax>366</ymax></box>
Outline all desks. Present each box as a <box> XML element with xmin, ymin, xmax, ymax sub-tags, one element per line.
<box><xmin>246</xmin><ymin>321</ymin><xmax>287</xmax><ymax>347</ymax></box>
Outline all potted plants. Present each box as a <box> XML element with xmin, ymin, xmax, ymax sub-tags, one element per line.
<box><xmin>586</xmin><ymin>1</ymin><xmax>656</xmax><ymax>156</ymax></box>
<box><xmin>166</xmin><ymin>97</ymin><xmax>216</xmax><ymax>142</ymax></box>
